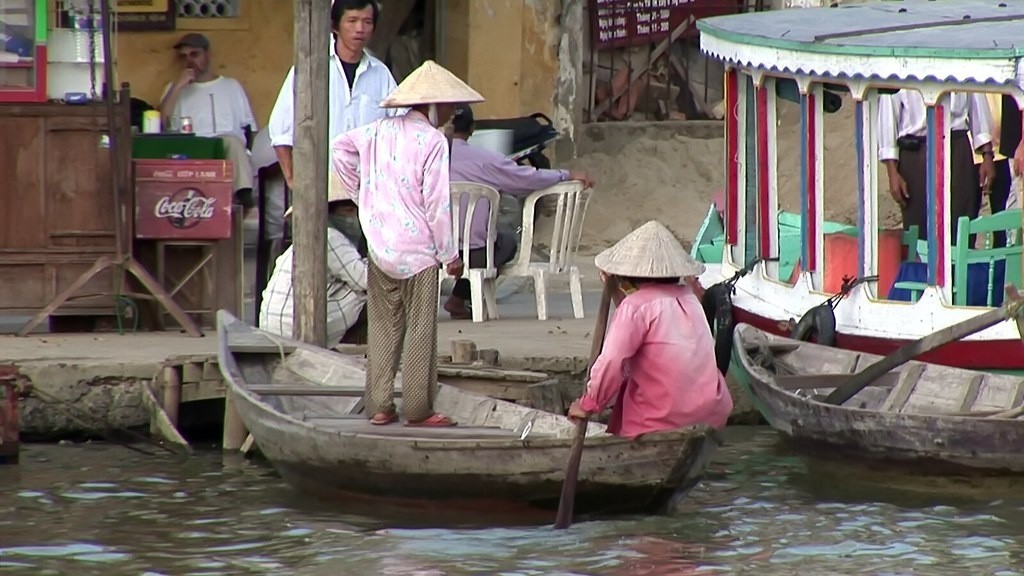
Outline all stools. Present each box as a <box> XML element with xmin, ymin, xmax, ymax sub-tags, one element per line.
<box><xmin>158</xmin><ymin>238</ymin><xmax>221</xmax><ymax>331</ymax></box>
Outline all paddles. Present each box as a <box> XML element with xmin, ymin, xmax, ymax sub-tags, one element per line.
<box><xmin>553</xmin><ymin>279</ymin><xmax>613</xmax><ymax>530</ymax></box>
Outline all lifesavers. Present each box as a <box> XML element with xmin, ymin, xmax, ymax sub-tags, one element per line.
<box><xmin>699</xmin><ymin>281</ymin><xmax>734</xmax><ymax>378</ymax></box>
<box><xmin>789</xmin><ymin>304</ymin><xmax>836</xmax><ymax>346</ymax></box>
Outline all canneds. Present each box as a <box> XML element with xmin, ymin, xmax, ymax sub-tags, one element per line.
<box><xmin>180</xmin><ymin>116</ymin><xmax>193</xmax><ymax>133</ymax></box>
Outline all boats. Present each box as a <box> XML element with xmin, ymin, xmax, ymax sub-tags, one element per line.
<box><xmin>690</xmin><ymin>0</ymin><xmax>1024</xmax><ymax>380</ymax></box>
<box><xmin>730</xmin><ymin>321</ymin><xmax>1024</xmax><ymax>475</ymax></box>
<box><xmin>217</xmin><ymin>309</ymin><xmax>724</xmax><ymax>511</ymax></box>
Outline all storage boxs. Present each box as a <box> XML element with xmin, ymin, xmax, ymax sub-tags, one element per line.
<box><xmin>134</xmin><ymin>157</ymin><xmax>235</xmax><ymax>239</ymax></box>
<box><xmin>131</xmin><ymin>135</ymin><xmax>227</xmax><ymax>159</ymax></box>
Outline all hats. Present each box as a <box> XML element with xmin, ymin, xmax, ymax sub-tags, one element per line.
<box><xmin>595</xmin><ymin>221</ymin><xmax>706</xmax><ymax>278</ymax></box>
<box><xmin>379</xmin><ymin>60</ymin><xmax>485</xmax><ymax>108</ymax></box>
<box><xmin>284</xmin><ymin>171</ymin><xmax>352</xmax><ymax>218</ymax></box>
<box><xmin>174</xmin><ymin>34</ymin><xmax>209</xmax><ymax>48</ymax></box>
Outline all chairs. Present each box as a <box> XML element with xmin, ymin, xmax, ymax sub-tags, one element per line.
<box><xmin>437</xmin><ymin>179</ymin><xmax>498</xmax><ymax>323</ymax></box>
<box><xmin>486</xmin><ymin>179</ymin><xmax>596</xmax><ymax>322</ymax></box>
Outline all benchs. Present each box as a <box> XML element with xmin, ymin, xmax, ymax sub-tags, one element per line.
<box><xmin>249</xmin><ymin>381</ymin><xmax>418</xmax><ymax>406</ymax></box>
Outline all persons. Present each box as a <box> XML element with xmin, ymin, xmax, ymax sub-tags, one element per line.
<box><xmin>567</xmin><ymin>219</ymin><xmax>734</xmax><ymax>442</ymax></box>
<box><xmin>878</xmin><ymin>87</ymin><xmax>1024</xmax><ymax>257</ymax></box>
<box><xmin>258</xmin><ymin>170</ymin><xmax>368</xmax><ymax>350</ymax></box>
<box><xmin>267</xmin><ymin>0</ymin><xmax>413</xmax><ymax>192</ymax></box>
<box><xmin>444</xmin><ymin>103</ymin><xmax>597</xmax><ymax>320</ymax></box>
<box><xmin>156</xmin><ymin>32</ymin><xmax>259</xmax><ymax>224</ymax></box>
<box><xmin>332</xmin><ymin>60</ymin><xmax>486</xmax><ymax>427</ymax></box>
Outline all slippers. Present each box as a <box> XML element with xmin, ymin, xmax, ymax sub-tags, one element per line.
<box><xmin>369</xmin><ymin>411</ymin><xmax>399</xmax><ymax>425</ymax></box>
<box><xmin>403</xmin><ymin>413</ymin><xmax>457</xmax><ymax>427</ymax></box>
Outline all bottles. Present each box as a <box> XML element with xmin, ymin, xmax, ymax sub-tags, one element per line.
<box><xmin>87</xmin><ymin>10</ymin><xmax>105</xmax><ymax>62</ymax></box>
<box><xmin>179</xmin><ymin>116</ymin><xmax>193</xmax><ymax>133</ymax></box>
<box><xmin>69</xmin><ymin>9</ymin><xmax>89</xmax><ymax>61</ymax></box>
<box><xmin>142</xmin><ymin>109</ymin><xmax>161</xmax><ymax>134</ymax></box>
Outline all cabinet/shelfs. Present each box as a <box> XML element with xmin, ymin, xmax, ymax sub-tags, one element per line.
<box><xmin>0</xmin><ymin>80</ymin><xmax>133</xmax><ymax>327</ymax></box>
